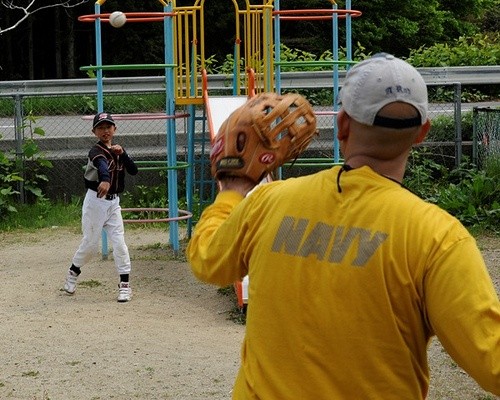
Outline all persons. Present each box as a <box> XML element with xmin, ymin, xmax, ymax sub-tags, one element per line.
<box><xmin>188</xmin><ymin>55</ymin><xmax>500</xmax><ymax>400</ymax></box>
<box><xmin>63</xmin><ymin>113</ymin><xmax>138</xmax><ymax>303</ymax></box>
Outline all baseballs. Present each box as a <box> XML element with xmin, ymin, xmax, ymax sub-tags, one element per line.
<box><xmin>109</xmin><ymin>12</ymin><xmax>126</xmax><ymax>27</ymax></box>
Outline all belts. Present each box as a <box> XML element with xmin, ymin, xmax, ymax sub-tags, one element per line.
<box><xmin>105</xmin><ymin>194</ymin><xmax>119</xmax><ymax>199</ymax></box>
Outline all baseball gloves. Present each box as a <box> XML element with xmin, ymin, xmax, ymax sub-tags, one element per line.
<box><xmin>210</xmin><ymin>92</ymin><xmax>317</xmax><ymax>181</ymax></box>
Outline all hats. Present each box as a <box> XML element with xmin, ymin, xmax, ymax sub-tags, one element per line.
<box><xmin>93</xmin><ymin>112</ymin><xmax>115</xmax><ymax>128</ymax></box>
<box><xmin>337</xmin><ymin>52</ymin><xmax>428</xmax><ymax>129</ymax></box>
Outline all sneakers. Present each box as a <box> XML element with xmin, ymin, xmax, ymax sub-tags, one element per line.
<box><xmin>118</xmin><ymin>282</ymin><xmax>132</xmax><ymax>301</ymax></box>
<box><xmin>64</xmin><ymin>266</ymin><xmax>78</xmax><ymax>294</ymax></box>
